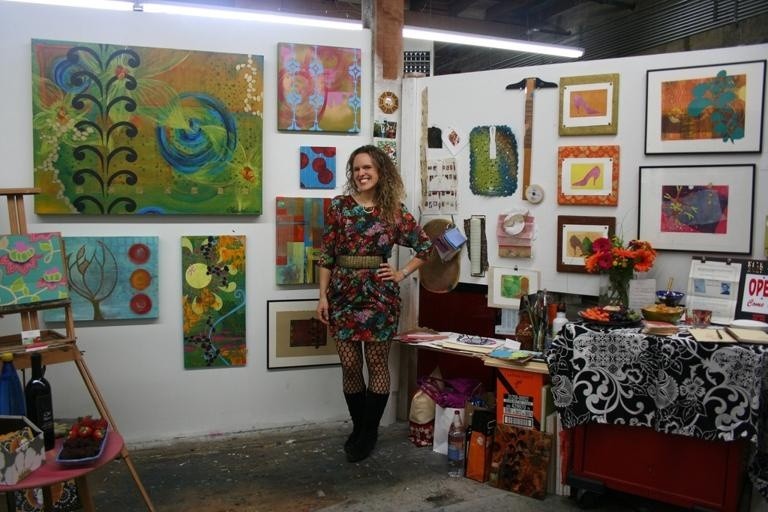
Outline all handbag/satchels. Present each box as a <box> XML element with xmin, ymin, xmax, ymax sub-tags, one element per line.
<box><xmin>432</xmin><ymin>373</ymin><xmax>497</xmax><ymax>484</ymax></box>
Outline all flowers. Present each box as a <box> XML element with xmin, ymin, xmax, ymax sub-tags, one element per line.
<box><xmin>582</xmin><ymin>235</ymin><xmax>657</xmax><ymax>308</ymax></box>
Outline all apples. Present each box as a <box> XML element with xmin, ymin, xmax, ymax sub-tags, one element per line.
<box><xmin>131</xmin><ymin>295</ymin><xmax>152</xmax><ymax>315</ymax></box>
<box><xmin>299</xmin><ymin>148</ymin><xmax>336</xmax><ymax>184</ymax></box>
<box><xmin>127</xmin><ymin>244</ymin><xmax>149</xmax><ymax>263</ymax></box>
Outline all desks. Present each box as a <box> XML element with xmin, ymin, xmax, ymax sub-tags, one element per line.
<box><xmin>0</xmin><ymin>417</ymin><xmax>124</xmax><ymax>512</ymax></box>
<box><xmin>392</xmin><ymin>334</ymin><xmax>554</xmax><ymax>433</ymax></box>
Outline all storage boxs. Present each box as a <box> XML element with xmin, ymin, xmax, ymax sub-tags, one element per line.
<box><xmin>495</xmin><ymin>366</ymin><xmax>545</xmax><ymax>431</ymax></box>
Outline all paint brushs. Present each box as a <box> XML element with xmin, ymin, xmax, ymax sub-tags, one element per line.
<box><xmin>523</xmin><ymin>288</ymin><xmax>550</xmax><ymax>335</ymax></box>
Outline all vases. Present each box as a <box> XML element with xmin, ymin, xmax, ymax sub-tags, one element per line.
<box><xmin>597</xmin><ymin>269</ymin><xmax>631</xmax><ymax>312</ymax></box>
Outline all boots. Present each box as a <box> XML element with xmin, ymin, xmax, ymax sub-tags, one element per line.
<box><xmin>342</xmin><ymin>385</ymin><xmax>390</xmax><ymax>464</ymax></box>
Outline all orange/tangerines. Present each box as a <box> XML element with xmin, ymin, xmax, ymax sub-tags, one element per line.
<box><xmin>130</xmin><ymin>268</ymin><xmax>151</xmax><ymax>291</ymax></box>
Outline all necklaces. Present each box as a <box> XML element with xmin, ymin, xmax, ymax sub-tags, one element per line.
<box><xmin>354</xmin><ymin>190</ymin><xmax>377</xmax><ymax>213</ymax></box>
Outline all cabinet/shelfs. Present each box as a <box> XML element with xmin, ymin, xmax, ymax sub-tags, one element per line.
<box><xmin>564</xmin><ymin>320</ymin><xmax>768</xmax><ymax>512</ymax></box>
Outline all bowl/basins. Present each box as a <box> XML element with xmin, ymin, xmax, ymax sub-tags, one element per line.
<box><xmin>640</xmin><ymin>304</ymin><xmax>686</xmax><ymax>325</ymax></box>
<box><xmin>655</xmin><ymin>290</ymin><xmax>685</xmax><ymax>307</ymax></box>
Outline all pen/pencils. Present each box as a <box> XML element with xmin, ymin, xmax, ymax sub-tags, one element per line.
<box><xmin>716</xmin><ymin>329</ymin><xmax>723</xmax><ymax>339</ymax></box>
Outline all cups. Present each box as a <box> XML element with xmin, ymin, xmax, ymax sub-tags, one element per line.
<box><xmin>691</xmin><ymin>309</ymin><xmax>712</xmax><ymax>329</ymax></box>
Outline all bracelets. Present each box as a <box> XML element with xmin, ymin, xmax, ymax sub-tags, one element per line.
<box><xmin>402</xmin><ymin>269</ymin><xmax>408</xmax><ymax>277</ymax></box>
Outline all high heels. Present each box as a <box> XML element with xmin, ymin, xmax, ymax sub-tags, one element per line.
<box><xmin>572</xmin><ymin>166</ymin><xmax>600</xmax><ymax>186</ymax></box>
<box><xmin>570</xmin><ymin>235</ymin><xmax>582</xmax><ymax>255</ymax></box>
<box><xmin>575</xmin><ymin>95</ymin><xmax>602</xmax><ymax>115</ymax></box>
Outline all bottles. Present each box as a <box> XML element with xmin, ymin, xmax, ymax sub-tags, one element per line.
<box><xmin>446</xmin><ymin>410</ymin><xmax>466</xmax><ymax>479</ymax></box>
<box><xmin>26</xmin><ymin>354</ymin><xmax>55</xmax><ymax>451</ymax></box>
<box><xmin>0</xmin><ymin>352</ymin><xmax>23</xmax><ymax>415</ymax></box>
<box><xmin>515</xmin><ymin>301</ymin><xmax>570</xmax><ymax>352</ymax></box>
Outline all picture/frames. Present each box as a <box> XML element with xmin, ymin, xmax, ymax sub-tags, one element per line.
<box><xmin>559</xmin><ymin>72</ymin><xmax>620</xmax><ymax>136</ymax></box>
<box><xmin>266</xmin><ymin>298</ymin><xmax>342</xmax><ymax>370</ymax></box>
<box><xmin>557</xmin><ymin>215</ymin><xmax>616</xmax><ymax>274</ymax></box>
<box><xmin>644</xmin><ymin>59</ymin><xmax>767</xmax><ymax>156</ymax></box>
<box><xmin>637</xmin><ymin>163</ymin><xmax>756</xmax><ymax>255</ymax></box>
<box><xmin>557</xmin><ymin>144</ymin><xmax>620</xmax><ymax>206</ymax></box>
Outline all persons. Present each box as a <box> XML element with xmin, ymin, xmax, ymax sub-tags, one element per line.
<box><xmin>315</xmin><ymin>145</ymin><xmax>432</xmax><ymax>463</ymax></box>
<box><xmin>721</xmin><ymin>281</ymin><xmax>729</xmax><ymax>294</ymax></box>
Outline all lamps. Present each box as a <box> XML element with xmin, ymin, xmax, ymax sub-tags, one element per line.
<box><xmin>0</xmin><ymin>0</ymin><xmax>586</xmax><ymax>59</ymax></box>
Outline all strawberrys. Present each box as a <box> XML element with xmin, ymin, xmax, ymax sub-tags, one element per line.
<box><xmin>66</xmin><ymin>415</ymin><xmax>107</xmax><ymax>441</ymax></box>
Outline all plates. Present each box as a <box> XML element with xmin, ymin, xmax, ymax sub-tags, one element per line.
<box><xmin>576</xmin><ymin>309</ymin><xmax>642</xmax><ymax>327</ymax></box>
<box><xmin>55</xmin><ymin>419</ymin><xmax>111</xmax><ymax>470</ymax></box>
<box><xmin>730</xmin><ymin>319</ymin><xmax>768</xmax><ymax>330</ymax></box>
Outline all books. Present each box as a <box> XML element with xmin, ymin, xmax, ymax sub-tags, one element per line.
<box><xmin>640</xmin><ymin>319</ymin><xmax>768</xmax><ymax>343</ymax></box>
<box><xmin>443</xmin><ymin>335</ymin><xmax>546</xmax><ymax>364</ymax></box>
